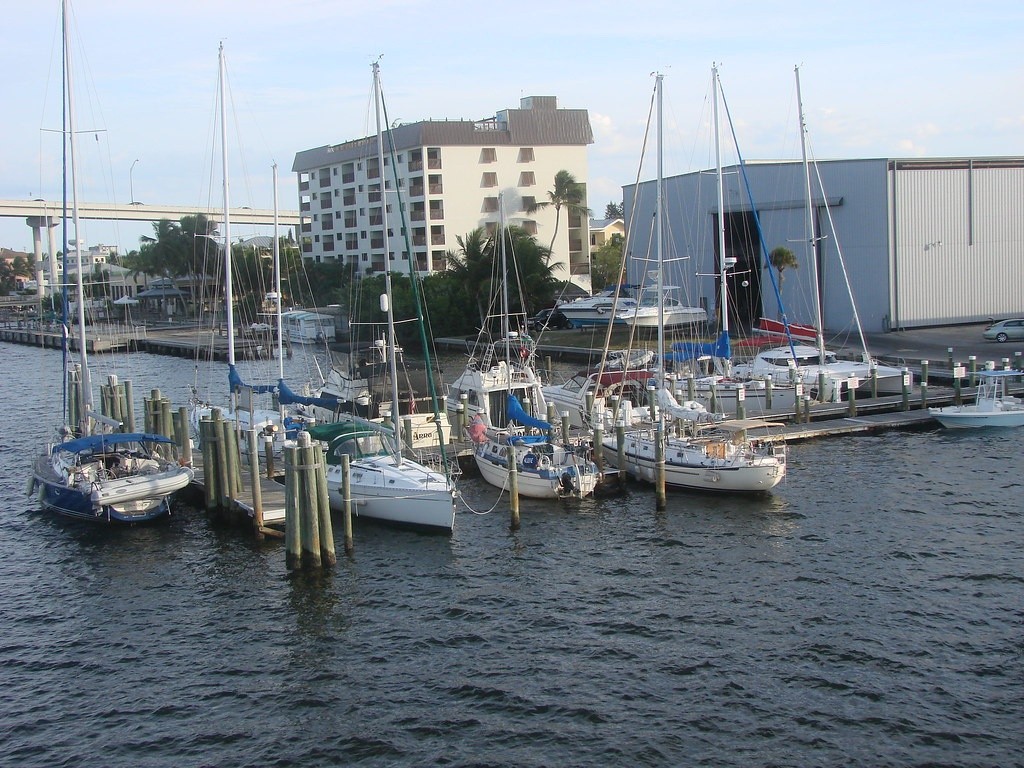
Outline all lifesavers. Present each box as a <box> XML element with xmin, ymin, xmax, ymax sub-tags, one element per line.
<box><xmin>536</xmin><ymin>454</ymin><xmax>552</xmax><ymax>471</ymax></box>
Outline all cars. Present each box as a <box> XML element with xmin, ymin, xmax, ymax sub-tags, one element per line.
<box><xmin>982</xmin><ymin>318</ymin><xmax>1024</xmax><ymax>343</ymax></box>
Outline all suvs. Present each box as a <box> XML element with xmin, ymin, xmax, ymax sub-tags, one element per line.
<box><xmin>525</xmin><ymin>306</ymin><xmax>573</xmax><ymax>332</ymax></box>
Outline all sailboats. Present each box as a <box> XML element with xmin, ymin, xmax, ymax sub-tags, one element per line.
<box><xmin>185</xmin><ymin>33</ymin><xmax>1024</xmax><ymax>537</ymax></box>
<box><xmin>28</xmin><ymin>1</ymin><xmax>196</xmax><ymax>531</ymax></box>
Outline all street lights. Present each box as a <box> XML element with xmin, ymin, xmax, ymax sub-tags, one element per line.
<box><xmin>129</xmin><ymin>157</ymin><xmax>139</xmax><ymax>206</ymax></box>
